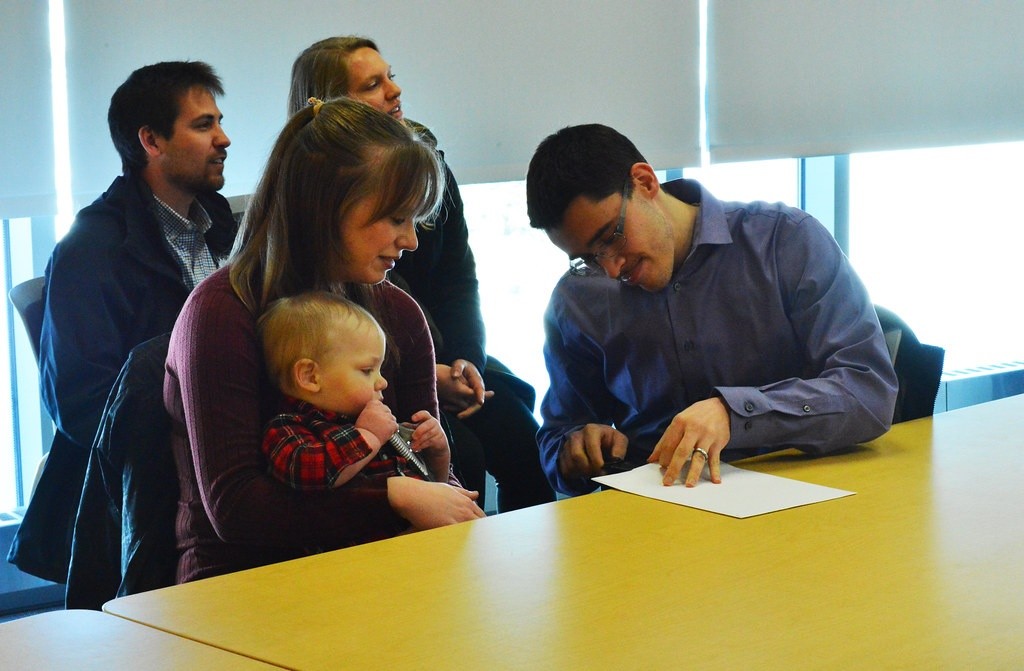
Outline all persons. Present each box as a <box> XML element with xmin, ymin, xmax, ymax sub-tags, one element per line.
<box><xmin>525</xmin><ymin>123</ymin><xmax>897</xmax><ymax>497</ymax></box>
<box><xmin>11</xmin><ymin>37</ymin><xmax>556</xmax><ymax>610</ymax></box>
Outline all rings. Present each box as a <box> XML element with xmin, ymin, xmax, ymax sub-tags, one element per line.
<box><xmin>695</xmin><ymin>447</ymin><xmax>708</xmax><ymax>456</ymax></box>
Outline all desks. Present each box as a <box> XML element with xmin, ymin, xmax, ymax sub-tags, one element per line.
<box><xmin>0</xmin><ymin>392</ymin><xmax>1024</xmax><ymax>671</ymax></box>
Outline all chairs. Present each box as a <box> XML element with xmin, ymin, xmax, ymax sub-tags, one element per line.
<box><xmin>64</xmin><ymin>332</ymin><xmax>179</xmax><ymax>610</ymax></box>
<box><xmin>873</xmin><ymin>303</ymin><xmax>945</xmax><ymax>425</ymax></box>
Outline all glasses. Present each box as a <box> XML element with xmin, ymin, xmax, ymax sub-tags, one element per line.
<box><xmin>569</xmin><ymin>178</ymin><xmax>630</xmax><ymax>277</ymax></box>
